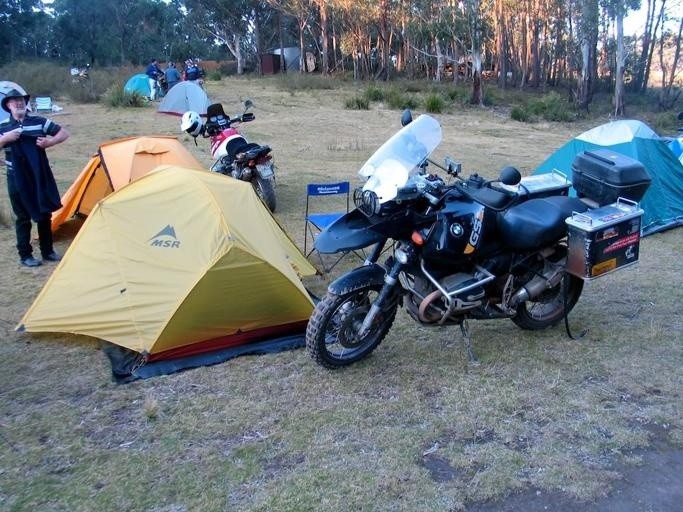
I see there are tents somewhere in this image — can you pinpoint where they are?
[527,119,682,238]
[123,73,158,100]
[50,133,206,237]
[157,80,213,117]
[0,80,31,123]
[10,164,343,384]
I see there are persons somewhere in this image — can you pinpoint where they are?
[144,57,205,101]
[0,89,71,268]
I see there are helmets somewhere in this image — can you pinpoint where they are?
[181,111,203,137]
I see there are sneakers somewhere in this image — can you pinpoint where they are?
[43,254,62,261]
[22,258,42,266]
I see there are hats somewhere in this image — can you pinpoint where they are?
[1,89,30,113]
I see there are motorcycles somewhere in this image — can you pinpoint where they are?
[306,109,650,373]
[71,64,92,86]
[181,99,282,213]
